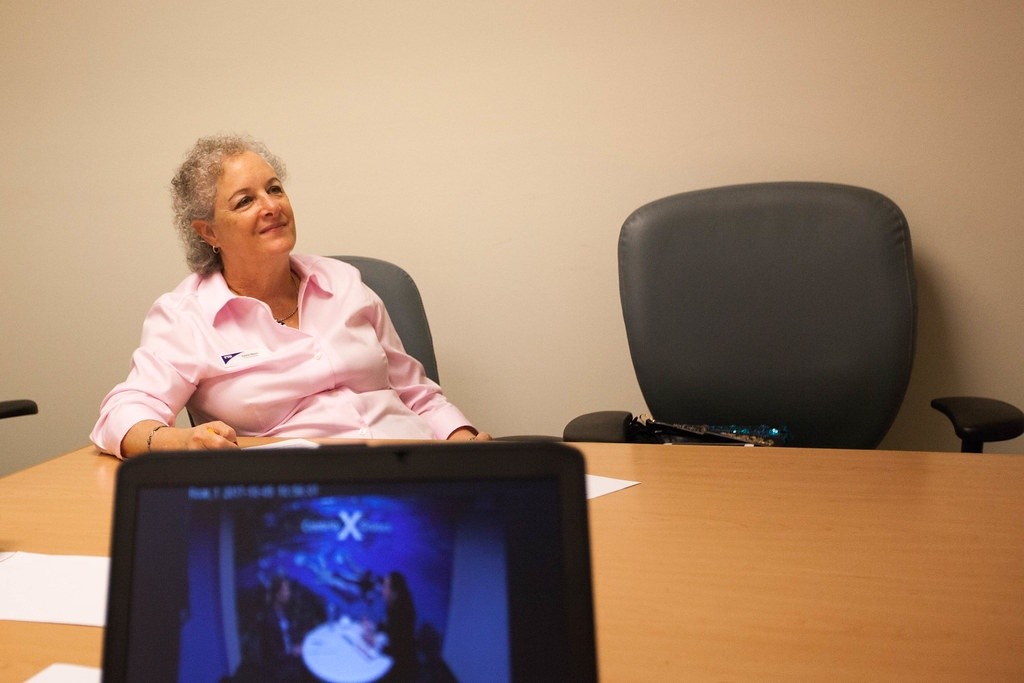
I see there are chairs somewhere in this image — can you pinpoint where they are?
[327,254,441,386]
[563,181,1024,454]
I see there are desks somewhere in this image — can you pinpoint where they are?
[0,443,1024,683]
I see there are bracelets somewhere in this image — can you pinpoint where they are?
[146,425,168,453]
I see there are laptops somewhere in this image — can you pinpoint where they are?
[100,439,600,682]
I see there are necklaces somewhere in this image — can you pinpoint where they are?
[272,306,298,326]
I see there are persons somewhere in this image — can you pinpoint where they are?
[262,576,304,683]
[90,135,493,462]
[366,572,418,682]
